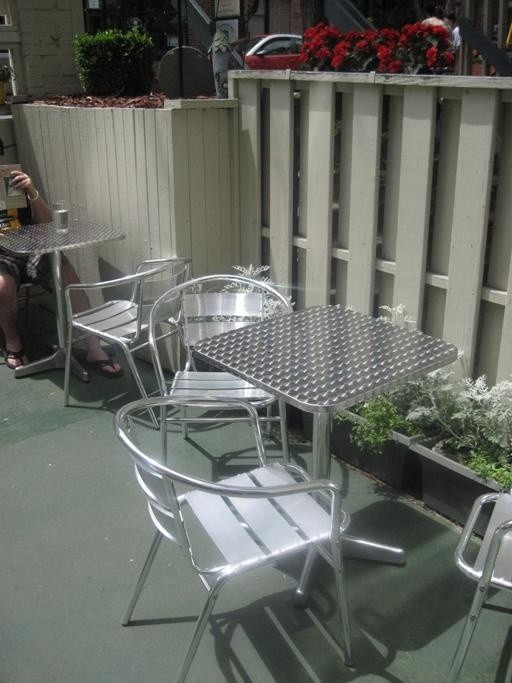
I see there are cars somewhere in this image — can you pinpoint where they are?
[238,33,308,101]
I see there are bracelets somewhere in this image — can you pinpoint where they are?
[27,188,38,201]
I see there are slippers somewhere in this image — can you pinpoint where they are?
[86,354,123,378]
[2,347,28,368]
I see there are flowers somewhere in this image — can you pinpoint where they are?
[299,23,455,73]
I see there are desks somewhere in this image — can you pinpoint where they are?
[191,304,459,612]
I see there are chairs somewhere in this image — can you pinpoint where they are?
[437,491,511,683]
[106,393,356,682]
[61,257,188,426]
[145,272,296,474]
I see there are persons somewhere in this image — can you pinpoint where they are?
[0,137,125,380]
[440,11,462,55]
[420,4,451,48]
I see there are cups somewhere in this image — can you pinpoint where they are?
[52,200,69,235]
